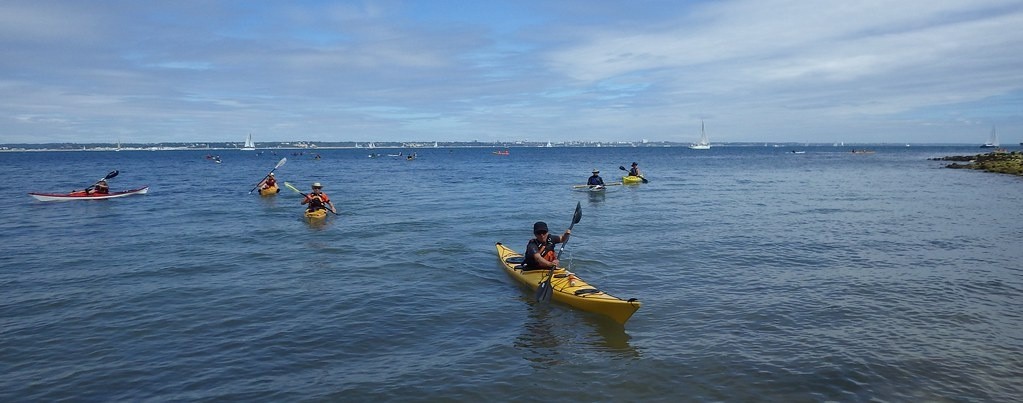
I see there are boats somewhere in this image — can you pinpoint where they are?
[26,184,149,202]
[258,184,280,197]
[216,159,223,165]
[371,155,417,161]
[495,241,644,327]
[303,202,331,223]
[494,152,511,155]
[586,183,609,194]
[623,174,646,185]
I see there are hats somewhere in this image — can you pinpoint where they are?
[312,182,320,186]
[269,173,275,176]
[534,223,548,231]
[631,162,638,165]
[591,168,600,172]
[99,178,106,182]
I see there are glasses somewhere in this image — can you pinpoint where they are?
[536,230,547,234]
[314,187,320,189]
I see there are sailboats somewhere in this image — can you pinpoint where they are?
[239,134,257,152]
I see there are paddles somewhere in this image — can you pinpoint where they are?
[534,200,583,307]
[618,165,649,182]
[573,182,622,189]
[283,182,341,216]
[248,157,287,195]
[72,169,120,193]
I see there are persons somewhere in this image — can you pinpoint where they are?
[588,169,603,185]
[301,183,336,213]
[408,154,412,159]
[399,151,402,156]
[523,222,570,271]
[628,162,639,176]
[95,178,109,194]
[216,156,220,161]
[258,172,276,188]
